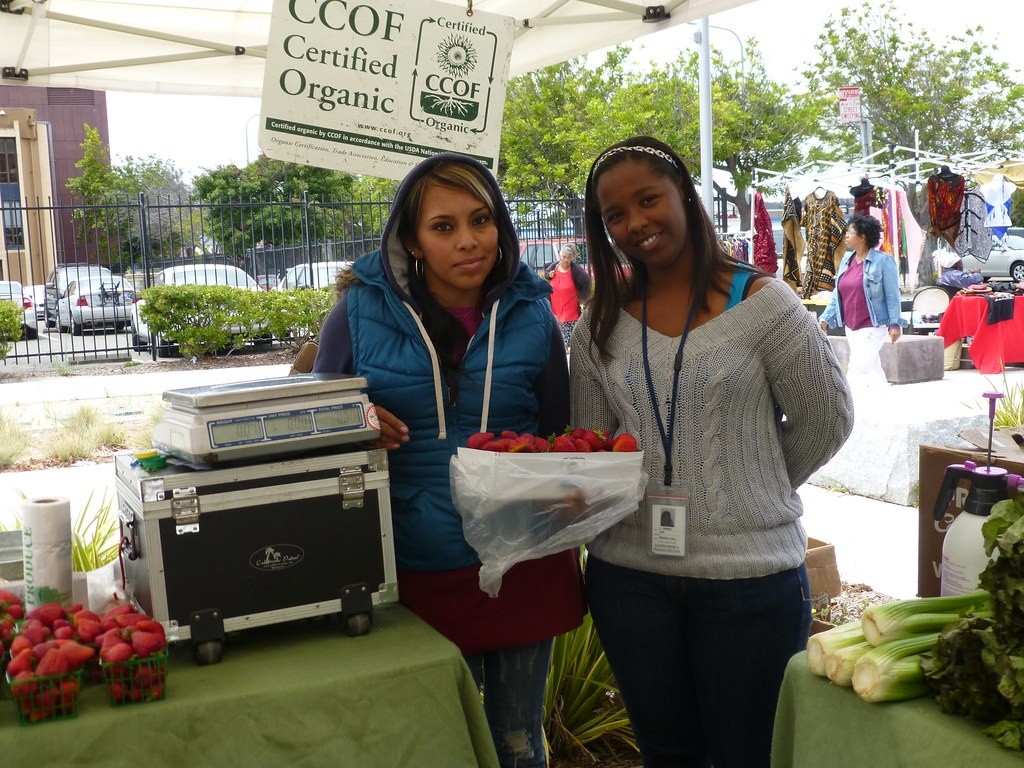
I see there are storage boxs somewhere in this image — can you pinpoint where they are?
[918,425,1024,599]
[113,448,400,667]
[457,446,644,501]
[804,537,841,604]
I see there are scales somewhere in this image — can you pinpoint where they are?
[148,371,382,466]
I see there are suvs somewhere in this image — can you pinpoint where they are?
[43,263,113,329]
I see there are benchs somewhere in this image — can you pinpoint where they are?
[824,334,945,385]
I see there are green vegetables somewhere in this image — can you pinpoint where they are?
[806,499,1024,754]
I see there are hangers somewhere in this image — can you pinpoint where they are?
[815,180,827,192]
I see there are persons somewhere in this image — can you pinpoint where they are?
[819,215,906,393]
[661,511,674,526]
[312,155,590,768]
[937,253,984,290]
[567,138,854,768]
[544,243,591,351]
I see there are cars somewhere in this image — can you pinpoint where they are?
[257,261,356,300]
[519,238,634,280]
[0,281,38,340]
[24,285,45,320]
[54,276,138,336]
[960,227,1024,283]
[130,263,274,358]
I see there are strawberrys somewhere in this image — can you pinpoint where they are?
[465,424,637,452]
[0,590,165,721]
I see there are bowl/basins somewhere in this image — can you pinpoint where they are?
[456,445,644,500]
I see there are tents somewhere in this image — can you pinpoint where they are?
[0,0,757,230]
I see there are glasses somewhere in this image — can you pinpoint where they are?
[846,229,858,236]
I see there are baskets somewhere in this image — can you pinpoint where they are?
[1,604,169,727]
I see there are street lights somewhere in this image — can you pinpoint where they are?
[686,20,744,97]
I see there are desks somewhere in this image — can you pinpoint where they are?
[936,295,1024,374]
[0,600,501,768]
[771,650,1024,768]
